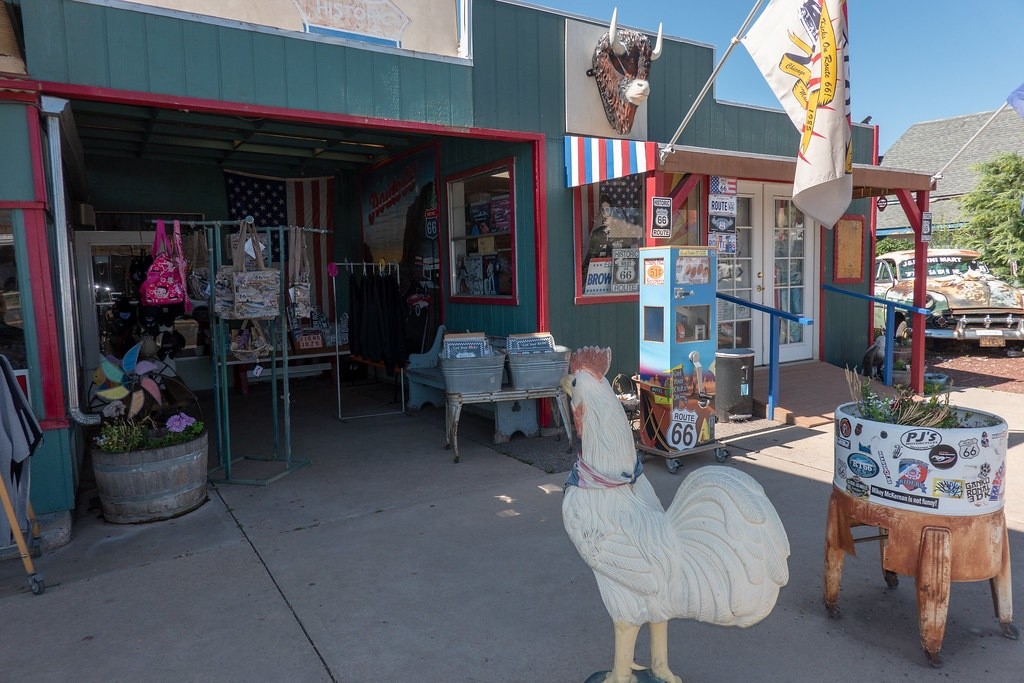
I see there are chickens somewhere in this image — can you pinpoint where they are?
[558,345,790,683]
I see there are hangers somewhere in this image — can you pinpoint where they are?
[363,262,367,276]
[380,263,382,276]
[373,262,375,274]
[393,261,397,271]
[388,262,391,275]
[350,263,354,273]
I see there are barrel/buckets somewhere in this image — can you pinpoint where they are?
[716,349,756,423]
[509,346,571,391]
[438,349,507,393]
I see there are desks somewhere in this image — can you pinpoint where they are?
[215,344,351,398]
[437,385,573,463]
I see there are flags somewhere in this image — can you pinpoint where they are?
[740,0,853,230]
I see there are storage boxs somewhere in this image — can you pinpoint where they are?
[438,350,505,393]
[499,345,571,391]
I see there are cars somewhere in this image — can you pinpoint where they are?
[873,248,1024,348]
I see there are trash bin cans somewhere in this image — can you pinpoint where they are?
[714,349,754,424]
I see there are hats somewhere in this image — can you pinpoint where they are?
[169,330,185,359]
[156,331,178,361]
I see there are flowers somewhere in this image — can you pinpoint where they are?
[166,413,195,433]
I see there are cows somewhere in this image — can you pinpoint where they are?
[592,7,663,136]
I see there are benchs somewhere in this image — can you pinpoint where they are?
[405,325,540,444]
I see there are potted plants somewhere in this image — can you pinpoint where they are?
[833,364,1008,516]
[149,419,150,420]
[892,361,912,387]
[92,421,209,524]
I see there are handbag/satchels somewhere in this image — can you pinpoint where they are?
[229,319,270,360]
[215,220,280,320]
[187,223,211,299]
[140,219,184,306]
[172,220,192,313]
[284,226,312,318]
